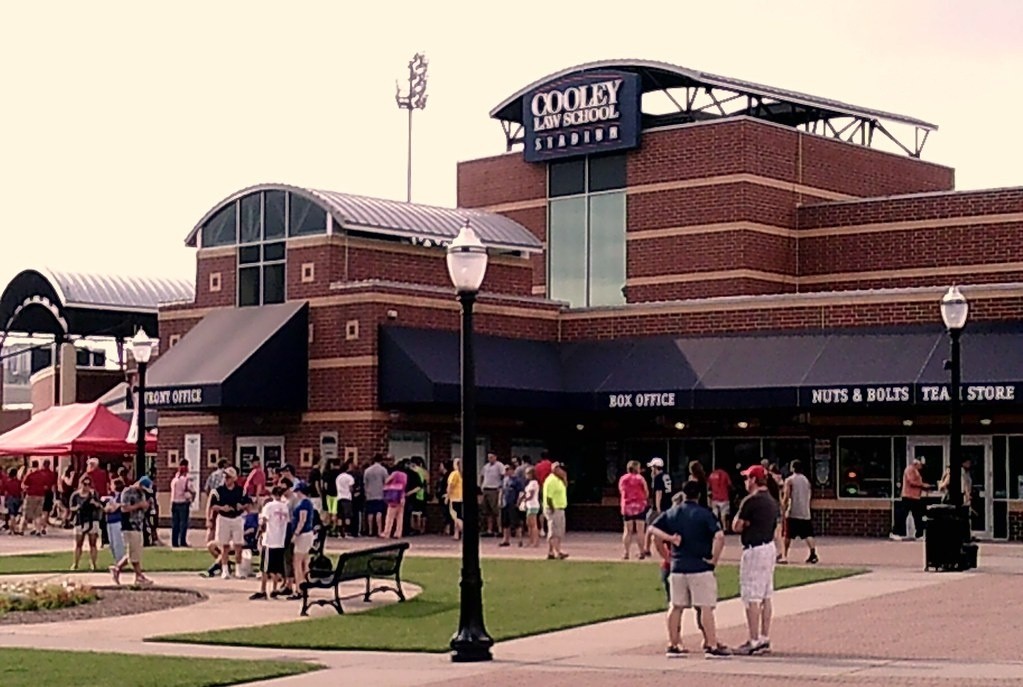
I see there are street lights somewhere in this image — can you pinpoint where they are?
[131,324,154,482]
[444,215,496,663]
[939,279,969,505]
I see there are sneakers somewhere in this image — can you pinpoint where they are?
[732,641,763,655]
[665,645,691,657]
[759,640,771,653]
[134,574,153,587]
[705,647,732,659]
[107,565,120,585]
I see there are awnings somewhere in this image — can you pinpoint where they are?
[378,321,1023,408]
[132,301,310,412]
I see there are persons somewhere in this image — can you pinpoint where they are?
[308,453,463,540]
[68,474,155,585]
[617,457,819,563]
[206,455,326,602]
[478,452,570,558]
[888,458,974,541]
[648,481,734,659]
[731,465,780,656]
[0,456,197,548]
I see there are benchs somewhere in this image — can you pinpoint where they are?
[299,542,410,615]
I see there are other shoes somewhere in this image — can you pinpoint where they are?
[559,553,568,559]
[10,530,96,571]
[172,542,308,601]
[548,554,556,559]
[806,555,818,563]
[499,542,509,546]
[539,529,546,537]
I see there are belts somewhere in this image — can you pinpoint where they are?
[745,541,768,548]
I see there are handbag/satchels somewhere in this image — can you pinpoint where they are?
[183,479,193,501]
[383,471,403,505]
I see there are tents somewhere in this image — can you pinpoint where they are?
[0,401,160,456]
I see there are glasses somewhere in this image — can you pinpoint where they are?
[84,482,91,486]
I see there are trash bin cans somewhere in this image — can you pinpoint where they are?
[925,504,959,567]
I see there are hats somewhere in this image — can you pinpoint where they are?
[740,465,767,479]
[289,481,307,493]
[243,455,259,462]
[646,457,664,467]
[139,476,154,494]
[222,467,237,480]
[682,481,700,496]
